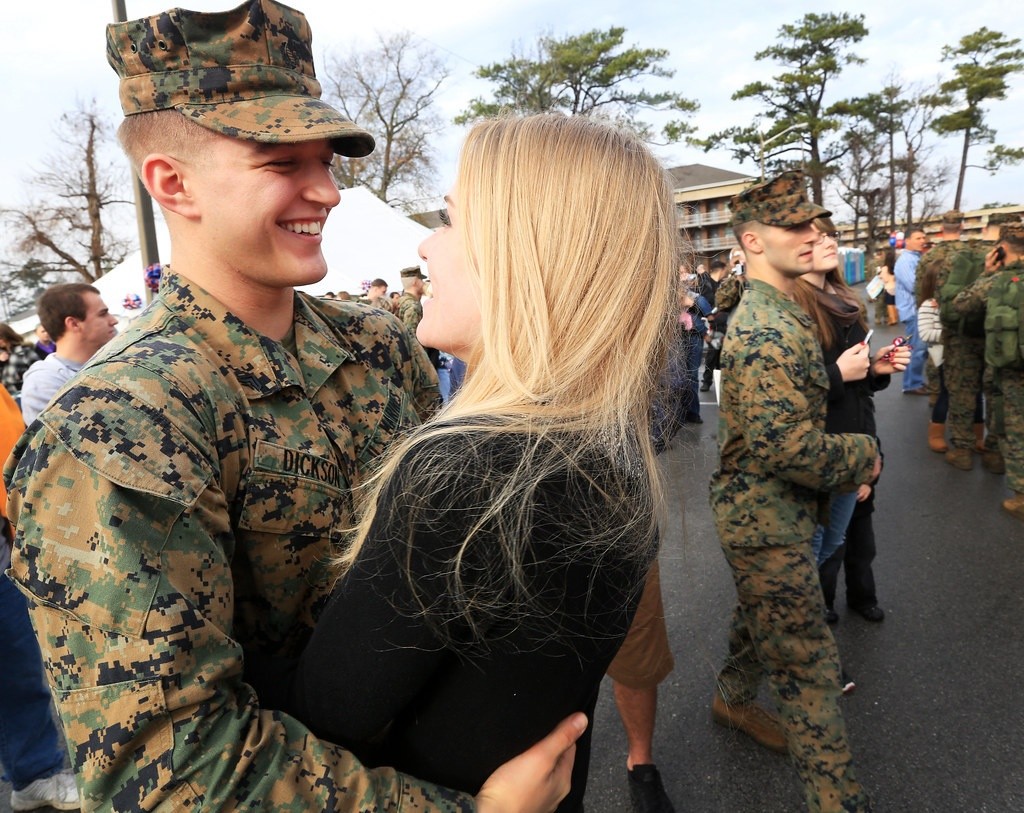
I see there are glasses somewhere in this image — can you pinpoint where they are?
[816,231,841,244]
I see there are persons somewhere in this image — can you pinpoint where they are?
[882,209,1024,520]
[277,114,681,813]
[646,260,727,457]
[0,0,588,813]
[707,170,912,813]
[606,554,674,813]
[324,266,427,335]
[0,283,119,813]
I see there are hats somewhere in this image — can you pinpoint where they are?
[106,0,375,158]
[728,170,832,225]
[943,210,964,223]
[987,214,1022,228]
[992,222,1024,245]
[400,268,427,279]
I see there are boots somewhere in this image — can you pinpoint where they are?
[927,420,948,452]
[974,421,985,454]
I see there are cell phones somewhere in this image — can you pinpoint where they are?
[995,246,1005,262]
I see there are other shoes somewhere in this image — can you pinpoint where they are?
[928,392,938,406]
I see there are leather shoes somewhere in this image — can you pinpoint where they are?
[847,601,884,621]
[922,382,930,389]
[903,384,932,395]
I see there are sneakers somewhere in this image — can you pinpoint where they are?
[945,446,974,472]
[626,764,675,813]
[1003,490,1024,520]
[712,695,788,754]
[982,446,1004,474]
